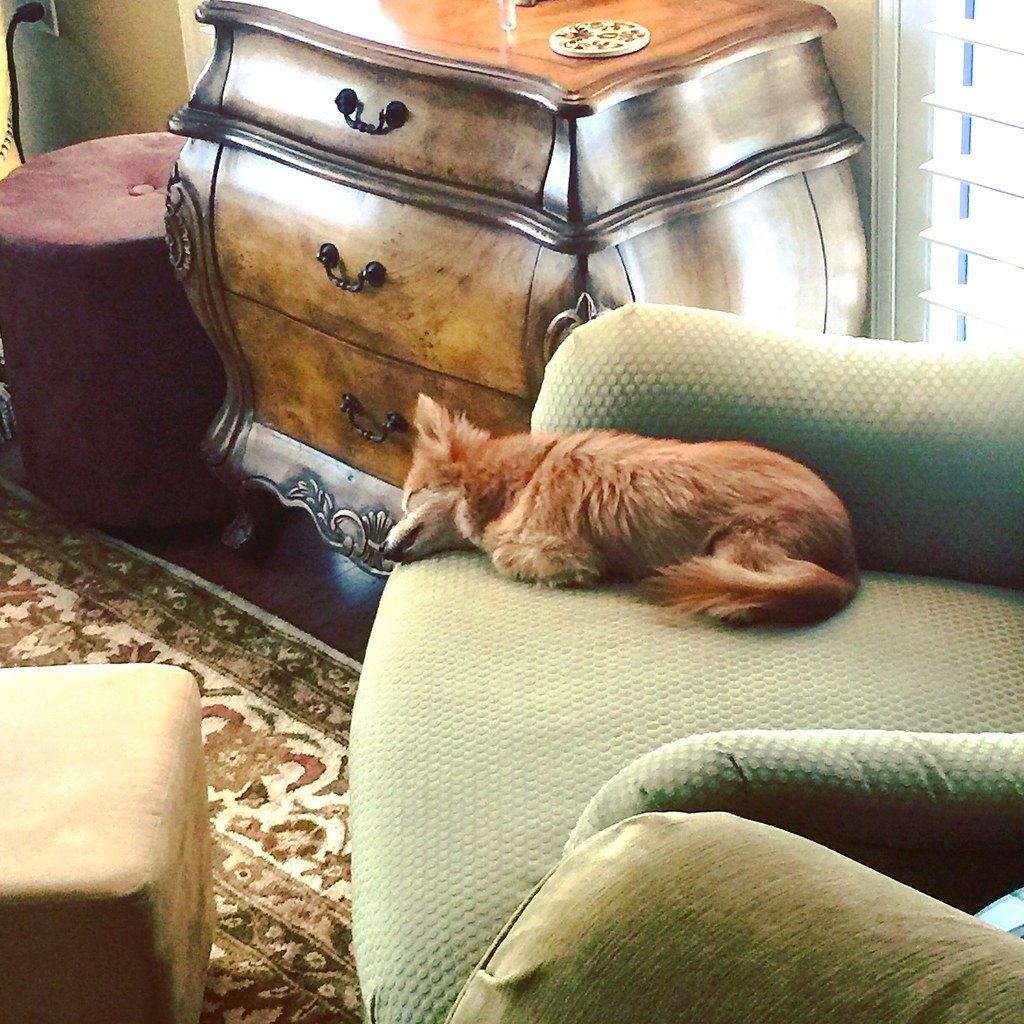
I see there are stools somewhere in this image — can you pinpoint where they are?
[0,664,215,1024]
[0,131,285,527]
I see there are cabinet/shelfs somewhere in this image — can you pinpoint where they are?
[165,0,876,579]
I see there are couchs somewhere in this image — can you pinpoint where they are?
[347,304,1022,1024]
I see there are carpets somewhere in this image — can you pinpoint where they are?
[0,472,360,1024]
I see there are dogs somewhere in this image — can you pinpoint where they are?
[379,390,861,629]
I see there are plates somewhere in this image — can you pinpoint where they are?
[550,20,651,59]
